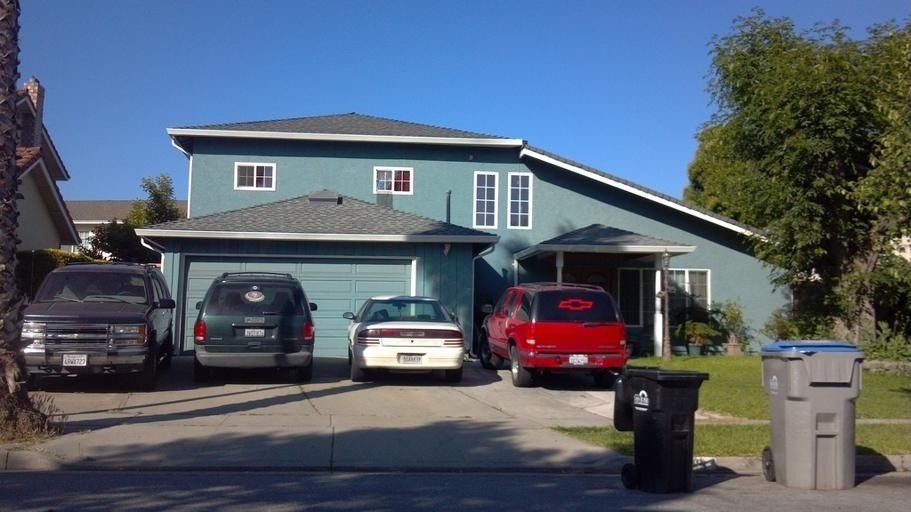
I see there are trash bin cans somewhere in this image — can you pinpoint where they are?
[614,365,709,493]
[760,339,865,490]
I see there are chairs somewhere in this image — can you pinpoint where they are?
[221,292,294,310]
[372,309,389,320]
[416,314,431,321]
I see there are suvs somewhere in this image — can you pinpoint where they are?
[475,280,629,387]
[192,270,318,386]
[16,261,173,386]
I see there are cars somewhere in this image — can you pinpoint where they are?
[342,294,466,382]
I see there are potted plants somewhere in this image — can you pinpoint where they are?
[674,298,745,357]
